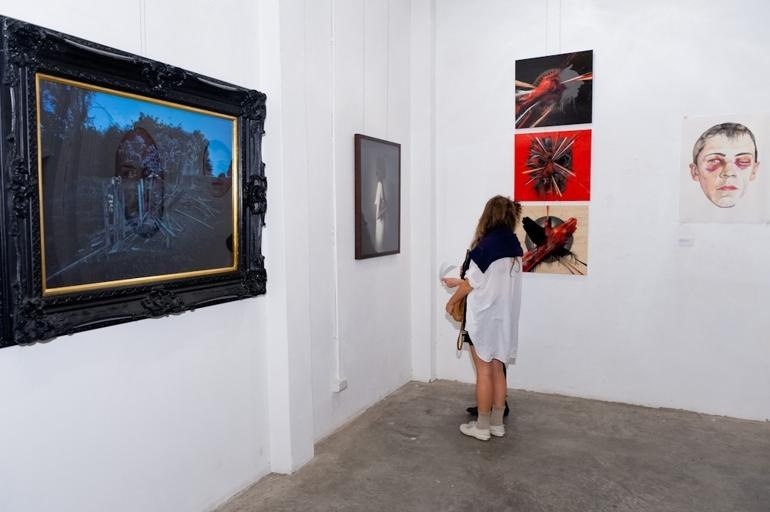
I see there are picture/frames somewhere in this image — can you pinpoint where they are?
[355,134,401,260]
[0,15,267,350]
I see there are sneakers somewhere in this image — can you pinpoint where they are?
[469,420,505,437]
[459,423,491,441]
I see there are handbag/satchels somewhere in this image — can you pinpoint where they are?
[451,296,465,321]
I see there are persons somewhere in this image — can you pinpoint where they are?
[372,154,389,252]
[110,127,168,239]
[444,194,525,442]
[688,122,761,210]
[440,199,521,418]
[201,138,234,199]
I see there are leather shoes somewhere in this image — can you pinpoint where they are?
[466,401,510,416]
